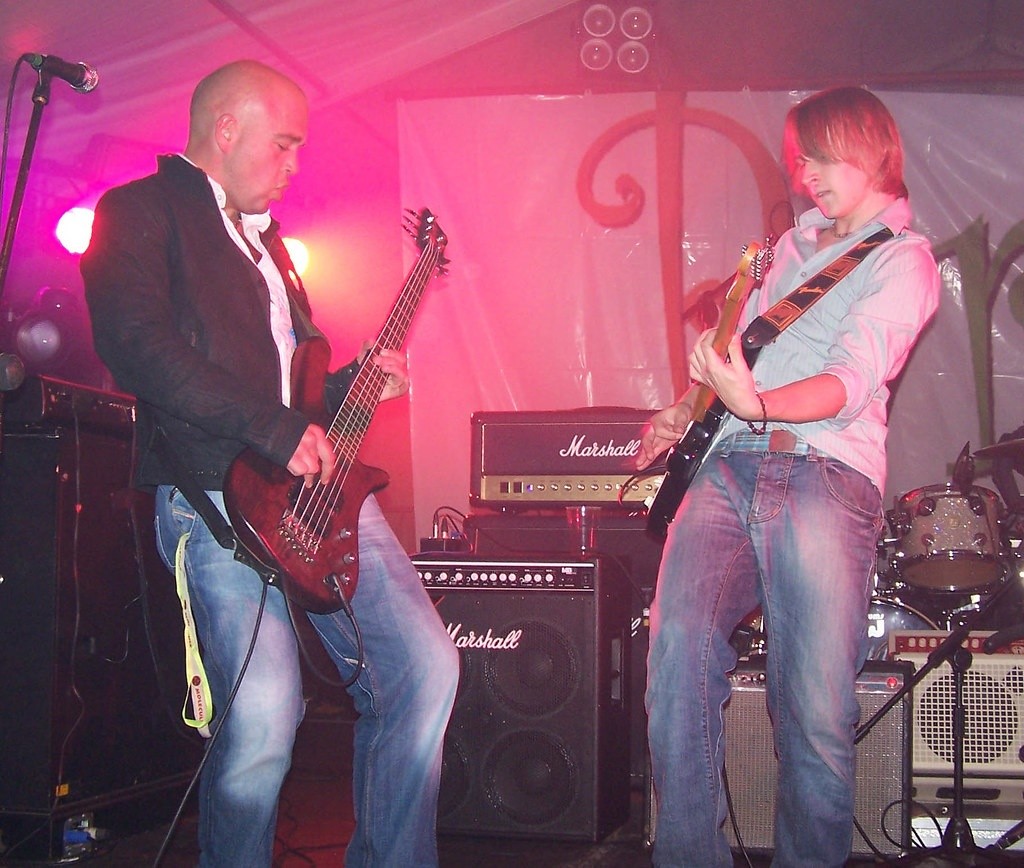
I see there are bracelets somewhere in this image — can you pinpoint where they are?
[747,395,767,435]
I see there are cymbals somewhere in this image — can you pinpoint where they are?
[972,436,1024,458]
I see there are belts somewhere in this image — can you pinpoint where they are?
[712,429,831,459]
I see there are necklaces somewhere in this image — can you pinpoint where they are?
[833,226,851,238]
[235,213,242,227]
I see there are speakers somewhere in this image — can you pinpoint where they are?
[641,658,914,864]
[461,514,667,610]
[410,551,629,842]
[886,628,1024,779]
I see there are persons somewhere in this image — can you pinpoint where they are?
[81,61,459,868]
[636,88,943,868]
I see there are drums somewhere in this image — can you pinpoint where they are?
[889,483,1011,597]
[856,596,942,672]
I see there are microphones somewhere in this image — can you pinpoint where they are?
[22,52,99,92]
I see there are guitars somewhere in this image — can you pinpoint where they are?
[220,204,451,617]
[643,234,774,539]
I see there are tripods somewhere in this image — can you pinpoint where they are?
[854,568,1024,868]
